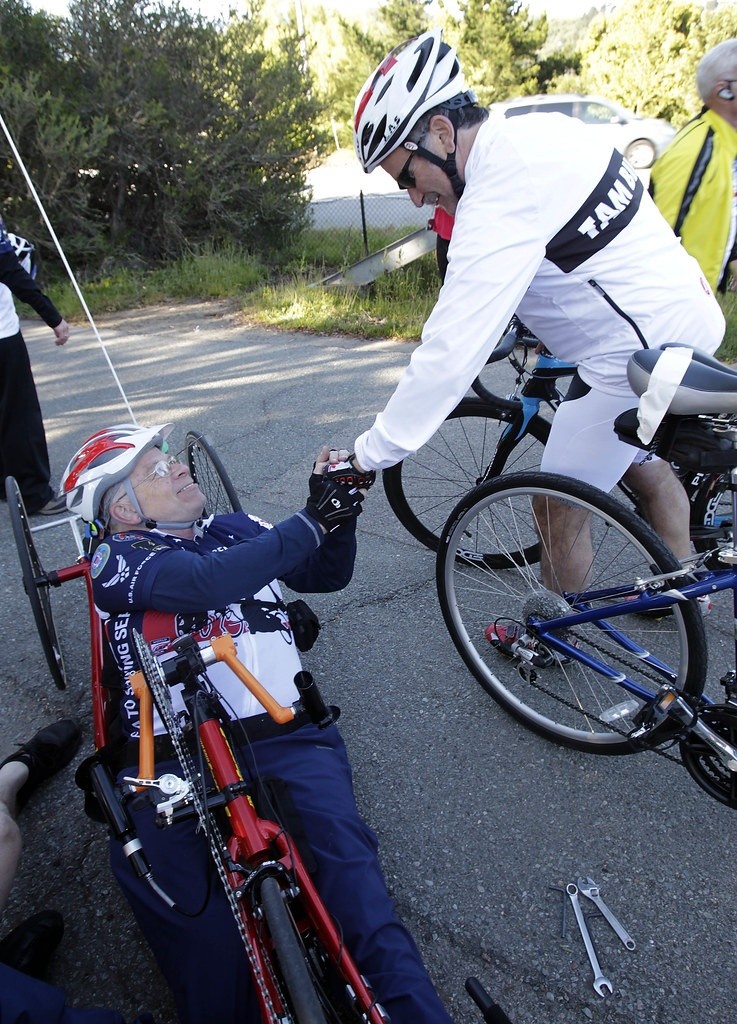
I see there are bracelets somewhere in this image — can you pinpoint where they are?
[344,451,376,490]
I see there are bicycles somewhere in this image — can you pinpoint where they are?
[6,430,510,1023]
[434,342,737,811]
[377,314,737,580]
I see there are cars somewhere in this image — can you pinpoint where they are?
[489,94,679,170]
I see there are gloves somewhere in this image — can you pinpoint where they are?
[322,453,375,492]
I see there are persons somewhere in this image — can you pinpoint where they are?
[1,219,78,522]
[648,37,736,353]
[1,716,132,1024]
[314,29,730,680]
[69,422,457,1024]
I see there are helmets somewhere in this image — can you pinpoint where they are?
[355,26,469,174]
[57,422,179,525]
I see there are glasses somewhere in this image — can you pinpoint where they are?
[115,454,185,503]
[397,123,431,190]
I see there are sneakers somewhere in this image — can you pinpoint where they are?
[38,490,68,515]
[484,621,575,667]
[0,909,64,980]
[0,719,80,819]
[623,588,713,620]
